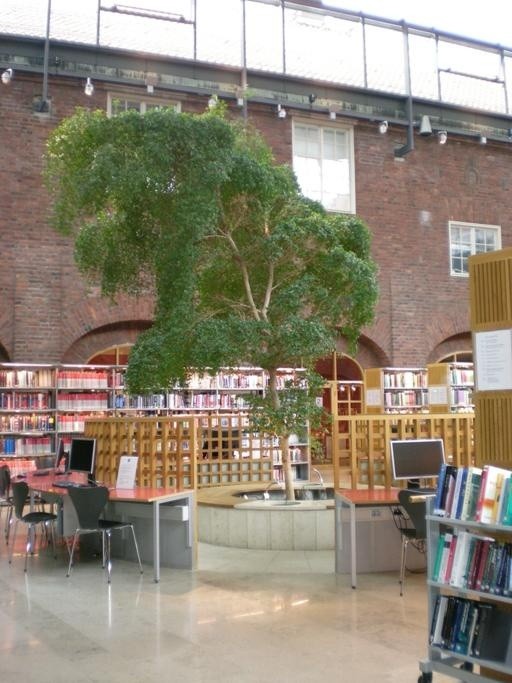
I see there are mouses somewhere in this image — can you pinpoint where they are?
[17,474,26,478]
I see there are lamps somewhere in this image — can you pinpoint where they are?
[0,66,489,147]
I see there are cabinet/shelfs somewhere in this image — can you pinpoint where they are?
[467,248,512,543]
[428,361,475,413]
[0,361,311,503]
[417,496,512,683]
[363,365,427,426]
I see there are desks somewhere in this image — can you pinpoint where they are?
[10,469,195,583]
[333,488,436,590]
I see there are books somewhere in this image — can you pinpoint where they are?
[57,368,113,468]
[1,368,57,489]
[384,368,475,426]
[211,418,305,480]
[114,368,305,417]
[426,462,512,660]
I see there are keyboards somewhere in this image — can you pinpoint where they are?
[33,469,49,476]
[52,480,92,488]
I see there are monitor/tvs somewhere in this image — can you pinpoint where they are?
[55,437,72,475]
[67,438,97,484]
[202,430,239,459]
[390,438,445,490]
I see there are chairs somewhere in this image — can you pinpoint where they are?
[0,465,145,584]
[399,490,437,596]
